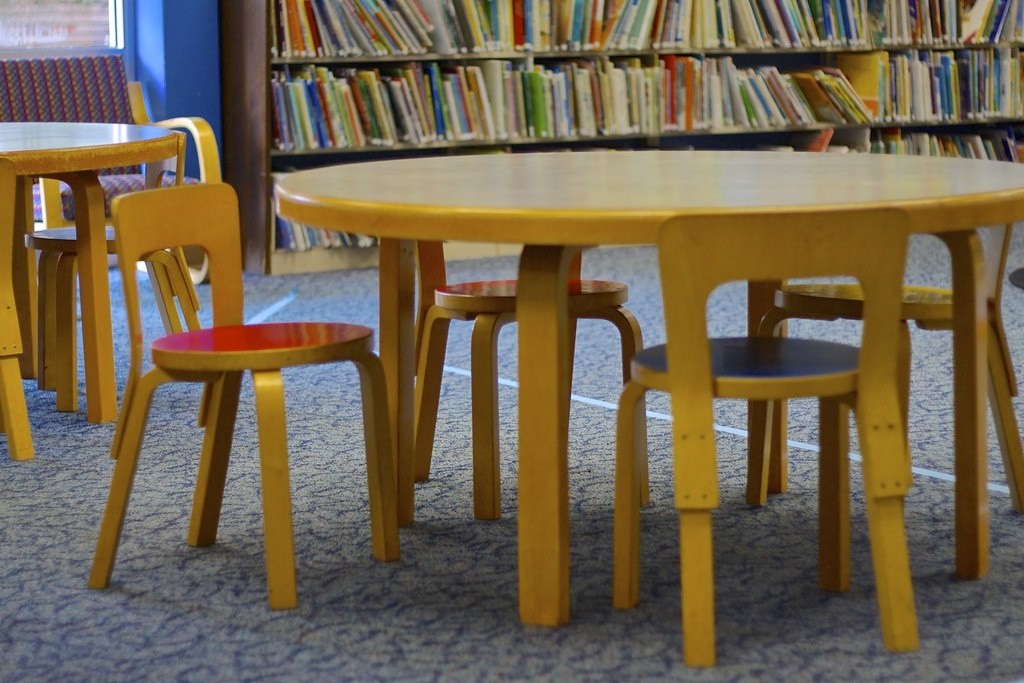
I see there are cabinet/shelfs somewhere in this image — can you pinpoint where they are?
[220,0,1024,275]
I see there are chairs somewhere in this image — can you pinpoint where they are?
[88,181,402,611]
[746,225,1024,513]
[613,206,921,653]
[25,131,202,425]
[415,238,649,522]
[0,159,34,462]
[0,54,222,284]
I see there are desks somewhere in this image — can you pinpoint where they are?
[0,122,179,426]
[274,150,1024,628]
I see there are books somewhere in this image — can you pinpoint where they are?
[268,0,1024,251]
